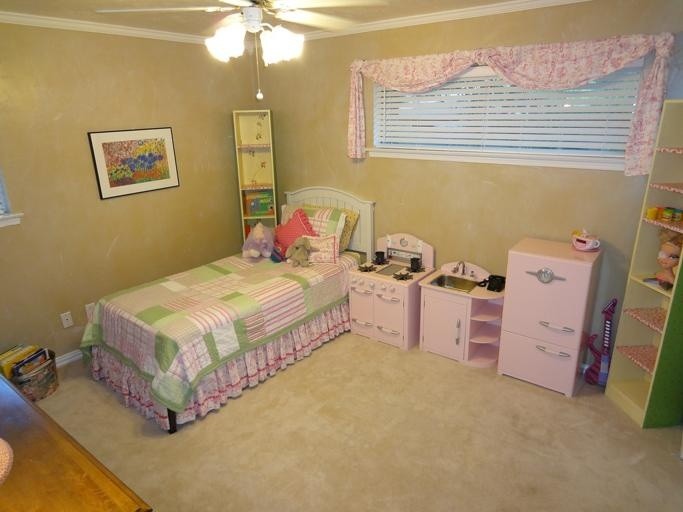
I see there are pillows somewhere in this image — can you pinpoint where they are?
[270,200,362,266]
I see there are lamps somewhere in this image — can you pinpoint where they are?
[199,5,310,67]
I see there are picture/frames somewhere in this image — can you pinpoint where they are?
[86,124,182,199]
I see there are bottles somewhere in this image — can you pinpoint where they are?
[645,206,681,224]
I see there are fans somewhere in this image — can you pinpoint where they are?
[95,0,390,36]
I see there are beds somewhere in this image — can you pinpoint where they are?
[81,185,376,435]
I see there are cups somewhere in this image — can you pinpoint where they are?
[410,257,420,271]
[488,274,504,292]
[375,252,385,264]
[572,235,601,250]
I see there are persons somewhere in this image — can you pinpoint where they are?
[657,227,683,292]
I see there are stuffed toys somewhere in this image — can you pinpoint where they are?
[241,221,276,258]
[285,236,311,267]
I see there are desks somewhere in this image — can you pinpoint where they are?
[0,373,155,512]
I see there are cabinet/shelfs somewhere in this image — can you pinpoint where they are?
[496,234,607,400]
[605,97,682,431]
[345,232,435,351]
[415,268,504,368]
[230,108,279,250]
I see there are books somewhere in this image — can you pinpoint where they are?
[0,344,50,379]
